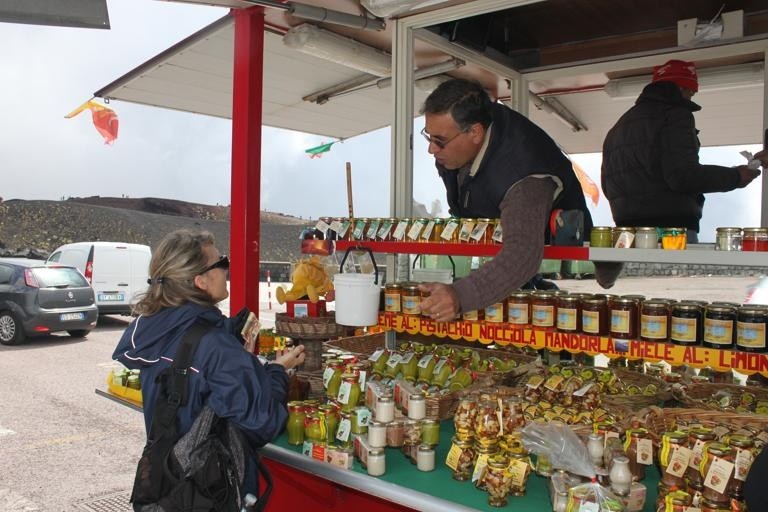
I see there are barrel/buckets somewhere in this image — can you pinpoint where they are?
[410,254,456,284]
[333,246,382,327]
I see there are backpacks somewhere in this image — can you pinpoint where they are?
[127,319,272,511]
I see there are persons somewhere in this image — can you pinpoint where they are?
[113,229,306,512]
[415,77,595,324]
[600,60,761,244]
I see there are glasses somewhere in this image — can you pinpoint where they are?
[199,254,230,275]
[421,123,469,149]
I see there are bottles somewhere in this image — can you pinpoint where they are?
[551,289,768,308]
[524,369,550,402]
[448,366,474,393]
[484,300,506,323]
[371,348,388,376]
[461,308,484,322]
[492,219,505,245]
[475,219,496,245]
[611,456,630,497]
[628,431,646,483]
[454,396,479,444]
[127,375,140,390]
[321,348,372,364]
[716,227,742,251]
[669,306,704,346]
[451,437,474,482]
[424,422,440,447]
[532,405,627,442]
[390,423,406,448]
[394,218,415,242]
[540,372,568,404]
[344,366,368,404]
[339,373,360,415]
[635,226,659,249]
[506,291,531,325]
[605,437,623,486]
[699,499,745,512]
[607,299,638,340]
[508,455,527,500]
[661,227,687,250]
[416,353,438,387]
[535,451,552,479]
[324,362,343,402]
[485,454,513,507]
[701,443,735,503]
[337,218,357,241]
[370,424,386,449]
[308,411,326,444]
[376,219,398,242]
[410,218,430,242]
[368,381,394,423]
[655,485,692,511]
[503,400,527,455]
[736,309,768,354]
[401,280,422,315]
[417,447,436,472]
[352,436,368,465]
[475,396,501,454]
[443,218,463,244]
[580,297,607,337]
[658,431,688,489]
[130,369,140,375]
[742,228,768,251]
[571,381,604,405]
[612,225,633,247]
[406,445,420,460]
[729,435,759,496]
[401,351,418,383]
[318,404,338,445]
[704,304,737,350]
[407,422,422,447]
[259,327,275,355]
[459,217,479,244]
[395,386,428,420]
[471,449,494,491]
[590,227,613,247]
[556,375,585,403]
[586,434,604,465]
[531,292,556,328]
[303,399,319,410]
[316,218,336,241]
[431,356,454,389]
[353,217,372,240]
[556,296,581,332]
[367,451,387,478]
[688,428,716,489]
[348,408,370,435]
[288,401,307,445]
[640,301,670,343]
[383,353,401,379]
[419,282,445,321]
[707,389,768,409]
[429,218,447,242]
[385,282,402,313]
[328,217,344,241]
[366,218,387,242]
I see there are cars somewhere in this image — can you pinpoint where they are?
[1,258,99,345]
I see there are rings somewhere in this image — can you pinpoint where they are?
[437,313,439,318]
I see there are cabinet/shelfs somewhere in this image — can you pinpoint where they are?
[94,242,768,512]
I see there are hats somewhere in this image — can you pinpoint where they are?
[652,61,698,93]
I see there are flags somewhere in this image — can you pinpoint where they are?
[64,100,118,145]
[304,143,333,159]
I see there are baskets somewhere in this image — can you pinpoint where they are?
[321,333,540,387]
[646,408,768,451]
[571,366,671,410]
[674,383,768,413]
[426,371,502,420]
[296,370,324,395]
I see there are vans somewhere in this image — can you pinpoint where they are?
[47,241,152,319]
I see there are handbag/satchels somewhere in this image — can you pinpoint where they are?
[131,323,241,511]
[275,313,347,337]
